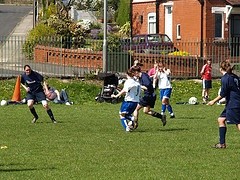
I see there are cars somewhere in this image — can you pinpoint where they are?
[123,33,175,54]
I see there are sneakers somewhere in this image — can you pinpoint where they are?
[202,97,207,104]
[161,115,167,125]
[52,119,56,123]
[126,122,137,131]
[170,113,175,118]
[212,143,226,148]
[31,117,37,123]
[161,112,165,115]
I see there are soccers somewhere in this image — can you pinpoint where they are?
[128,121,138,130]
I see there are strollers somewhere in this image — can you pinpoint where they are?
[96,71,121,103]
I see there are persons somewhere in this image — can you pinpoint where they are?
[156,62,175,118]
[20,64,74,123]
[111,60,167,132]
[201,57,213,104]
[206,60,240,148]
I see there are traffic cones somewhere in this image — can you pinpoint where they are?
[9,74,22,103]
